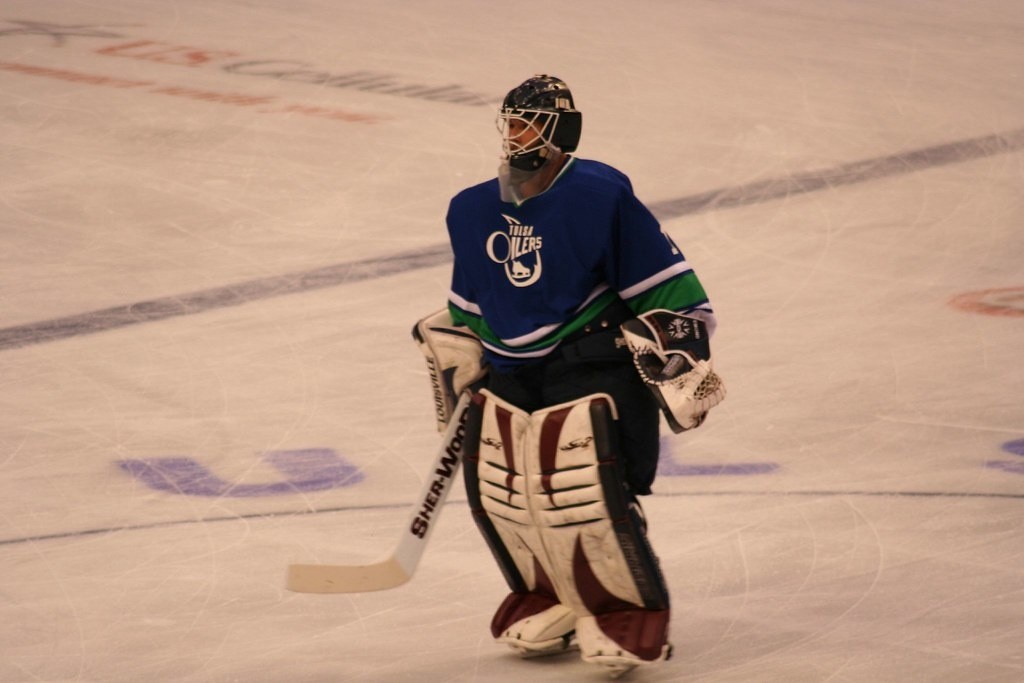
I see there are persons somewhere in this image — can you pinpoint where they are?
[412,74,723,678]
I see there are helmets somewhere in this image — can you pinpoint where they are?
[494,75,583,172]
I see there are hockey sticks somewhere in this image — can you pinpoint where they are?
[280,385,481,598]
